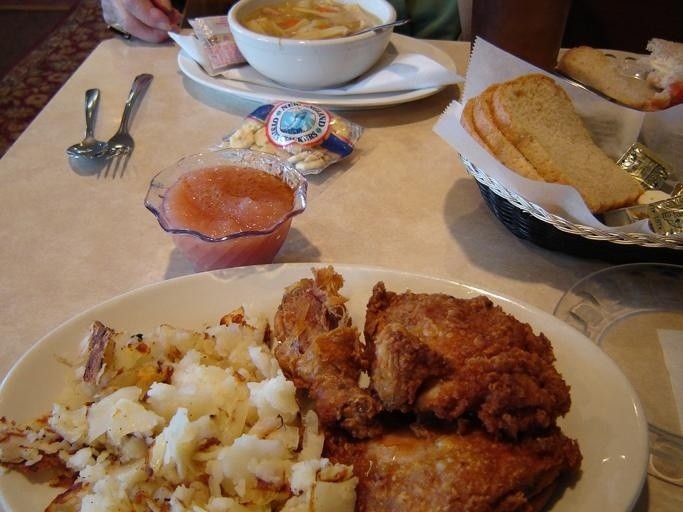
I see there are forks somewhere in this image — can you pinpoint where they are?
[101,74,151,159]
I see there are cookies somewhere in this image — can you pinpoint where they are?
[219,115,350,170]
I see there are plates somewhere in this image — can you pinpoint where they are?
[553,262,683,462]
[175,31,457,114]
[0,258,649,511]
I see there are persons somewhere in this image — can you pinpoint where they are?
[101,0,475,42]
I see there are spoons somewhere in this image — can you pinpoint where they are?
[68,88,107,157]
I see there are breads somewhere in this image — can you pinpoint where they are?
[461,37,682,218]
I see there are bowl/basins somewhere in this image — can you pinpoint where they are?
[143,151,313,269]
[226,2,400,95]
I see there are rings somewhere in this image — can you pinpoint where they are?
[112,24,127,33]
